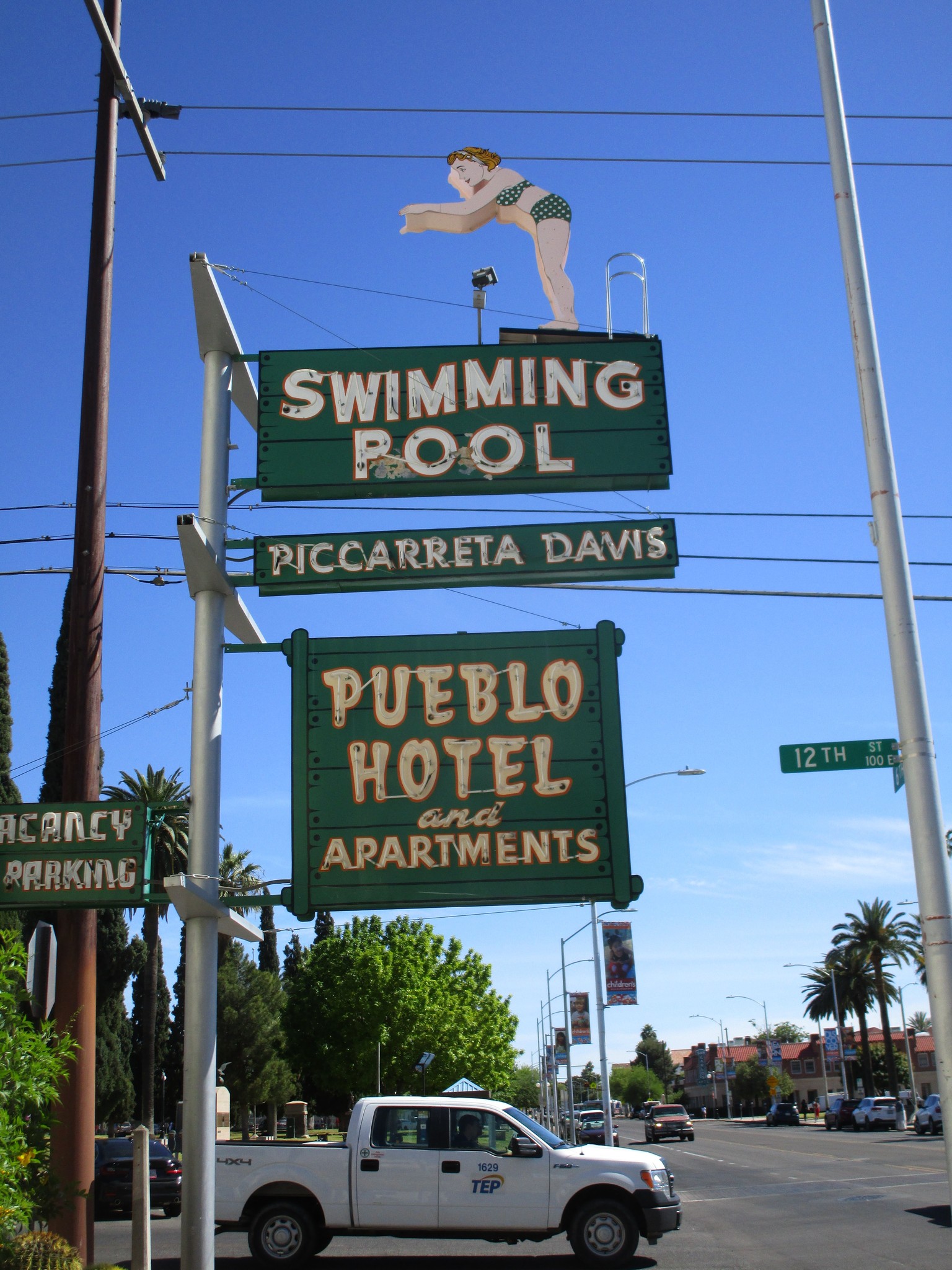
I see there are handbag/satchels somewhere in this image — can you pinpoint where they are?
[702,1111,703,1114]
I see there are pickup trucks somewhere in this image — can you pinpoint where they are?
[215,1096,684,1270]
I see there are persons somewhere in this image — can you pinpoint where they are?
[336,1117,339,1128]
[700,1105,707,1118]
[801,1099,810,1121]
[895,1097,906,1131]
[452,1114,483,1148]
[813,1097,820,1123]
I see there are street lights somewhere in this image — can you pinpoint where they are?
[560,908,640,1145]
[536,1010,571,1128]
[782,962,849,1101]
[159,1070,167,1139]
[572,1081,583,1104]
[589,766,705,1147]
[897,982,919,1106]
[689,1014,731,1119]
[546,957,602,1139]
[726,995,776,1104]
[657,1040,668,1105]
[584,1074,599,1100]
[625,1050,651,1102]
[706,1071,717,1119]
[577,1078,589,1101]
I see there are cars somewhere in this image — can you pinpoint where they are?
[17,1136,183,1218]
[532,1099,660,1147]
[106,1122,133,1138]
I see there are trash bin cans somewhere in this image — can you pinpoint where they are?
[714,1113,720,1119]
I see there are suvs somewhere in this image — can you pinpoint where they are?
[824,1099,863,1131]
[913,1094,943,1136]
[766,1102,800,1127]
[644,1104,695,1144]
[851,1096,898,1133]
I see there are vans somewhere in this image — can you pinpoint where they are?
[808,1092,848,1113]
